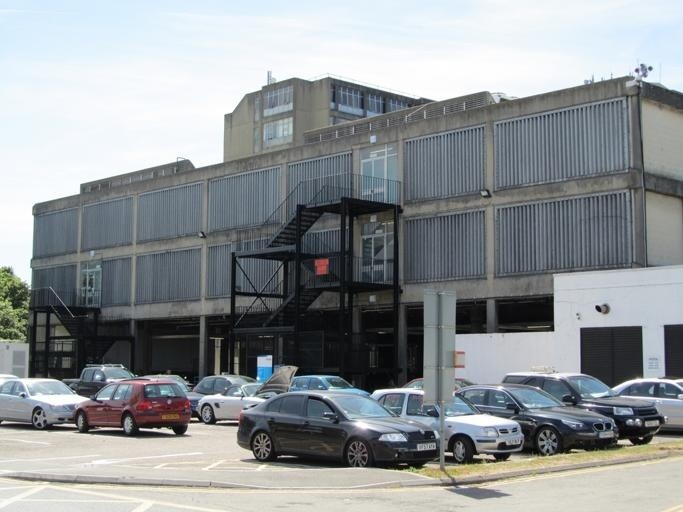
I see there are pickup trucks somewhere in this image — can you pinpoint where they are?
[60,362,136,396]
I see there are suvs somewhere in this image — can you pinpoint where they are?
[502,370,668,448]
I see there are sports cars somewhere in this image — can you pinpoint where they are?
[194,364,299,425]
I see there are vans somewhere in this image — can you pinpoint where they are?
[366,387,528,466]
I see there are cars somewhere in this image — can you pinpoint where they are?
[140,373,205,421]
[70,377,193,437]
[236,387,440,469]
[0,376,91,431]
[453,381,617,457]
[386,375,477,401]
[582,377,683,434]
[0,373,19,385]
[289,374,371,397]
[191,372,262,396]
